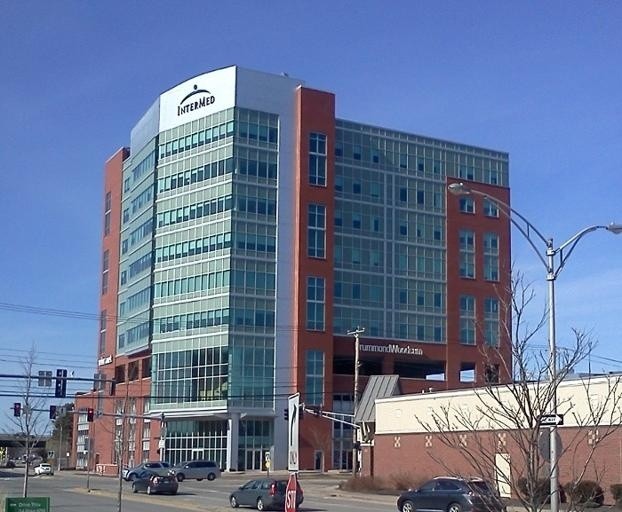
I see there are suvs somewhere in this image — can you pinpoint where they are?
[230,479,304,511]
[123,461,172,482]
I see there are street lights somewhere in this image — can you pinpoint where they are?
[445,182,622,512]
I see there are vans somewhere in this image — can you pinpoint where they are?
[170,461,220,481]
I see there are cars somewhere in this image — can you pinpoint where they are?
[131,471,178,494]
[396,474,506,511]
[34,463,53,475]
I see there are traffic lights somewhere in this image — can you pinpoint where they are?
[86,407,94,422]
[283,408,288,420]
[14,403,20,417]
[48,405,56,420]
